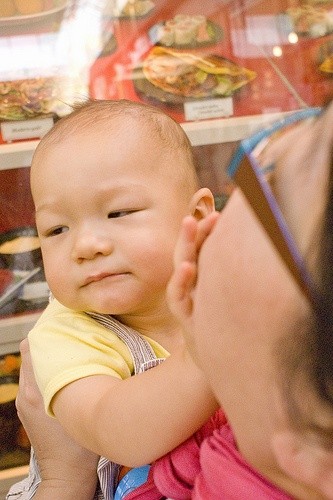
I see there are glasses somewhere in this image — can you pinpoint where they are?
[225,108,315,310]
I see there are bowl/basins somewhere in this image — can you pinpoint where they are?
[0,226,44,272]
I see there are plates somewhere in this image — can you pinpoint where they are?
[0,267,40,310]
[146,19,223,49]
[134,44,242,106]
[17,280,53,305]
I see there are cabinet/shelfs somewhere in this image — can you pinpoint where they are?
[0,2,333,500]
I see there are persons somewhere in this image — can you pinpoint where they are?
[13,93,333,500]
[0,99,223,500]
